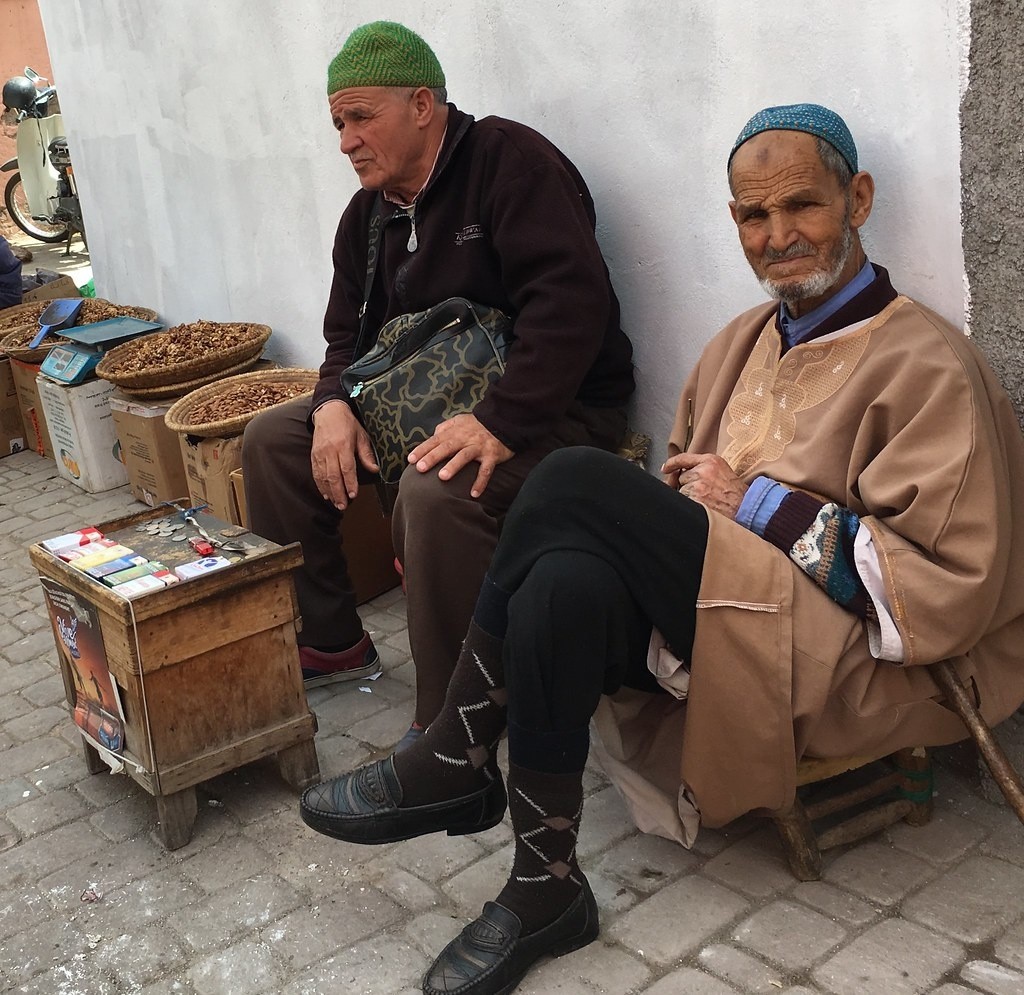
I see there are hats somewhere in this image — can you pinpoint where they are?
[728,104,859,175]
[327,21,445,95]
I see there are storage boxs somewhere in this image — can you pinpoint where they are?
[178,431,247,528]
[22,268,82,305]
[35,373,129,493]
[109,393,190,507]
[0,353,28,458]
[10,356,56,461]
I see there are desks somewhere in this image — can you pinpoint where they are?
[30,498,322,852]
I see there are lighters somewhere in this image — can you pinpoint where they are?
[187,533,216,556]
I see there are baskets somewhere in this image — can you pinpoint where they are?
[0,298,157,363]
[164,367,319,437]
[96,322,271,401]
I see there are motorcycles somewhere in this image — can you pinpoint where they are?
[0,66,89,258]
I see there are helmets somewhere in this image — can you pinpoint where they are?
[3,76,36,109]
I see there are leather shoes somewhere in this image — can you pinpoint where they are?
[422,873,600,995]
[300,751,508,845]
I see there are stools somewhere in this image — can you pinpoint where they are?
[772,741,934,880]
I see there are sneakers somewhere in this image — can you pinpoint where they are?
[299,630,381,690]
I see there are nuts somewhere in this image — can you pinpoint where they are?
[0,297,150,349]
[111,318,260,374]
[187,381,308,425]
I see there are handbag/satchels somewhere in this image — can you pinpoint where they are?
[340,297,517,487]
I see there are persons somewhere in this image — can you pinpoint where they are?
[294,97,1023,994]
[238,16,639,762]
